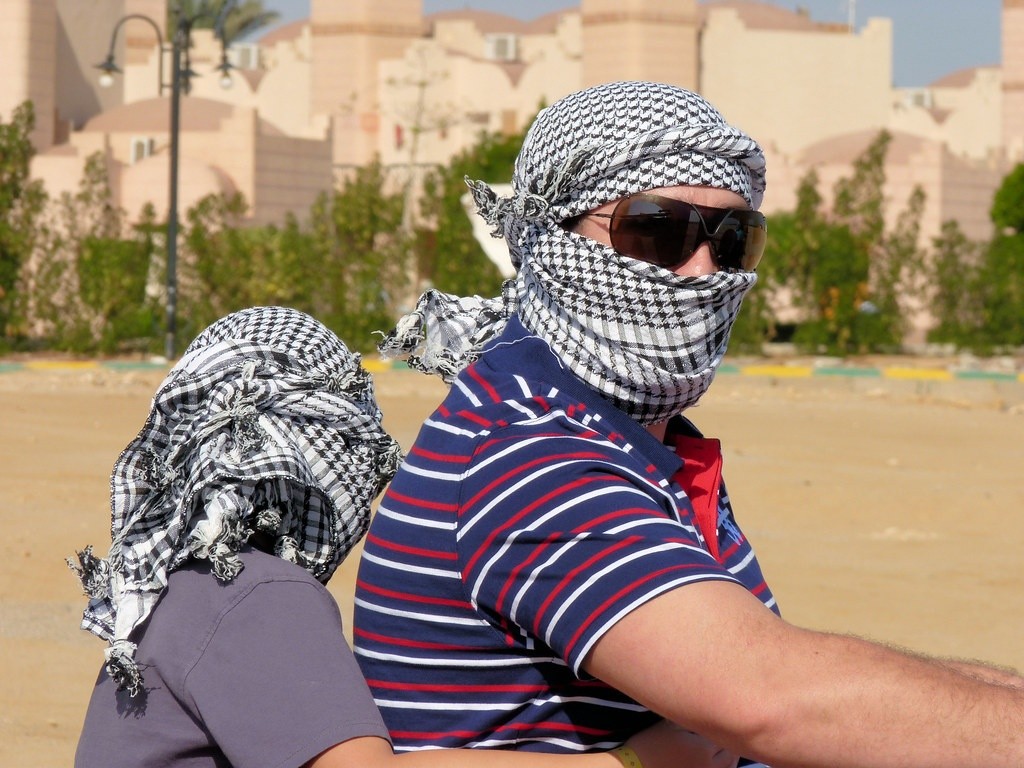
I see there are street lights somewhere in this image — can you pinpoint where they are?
[94,11,236,368]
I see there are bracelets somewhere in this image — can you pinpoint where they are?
[610,745,642,768]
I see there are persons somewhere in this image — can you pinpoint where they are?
[63,305,741,768]
[352,80,1024,768]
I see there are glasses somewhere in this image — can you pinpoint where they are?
[577,193,770,276]
